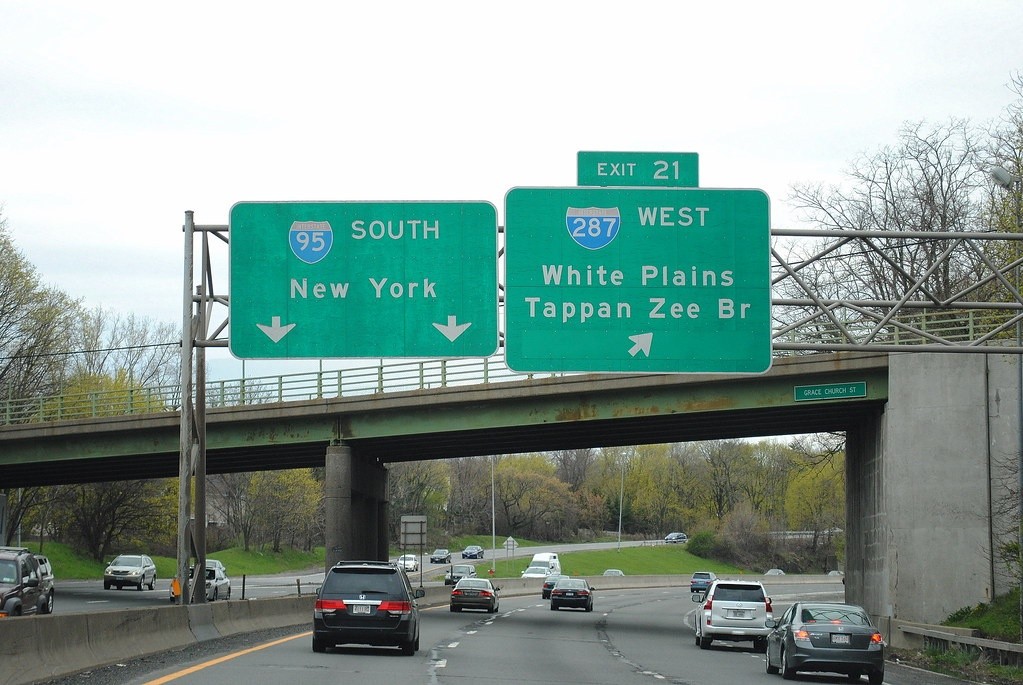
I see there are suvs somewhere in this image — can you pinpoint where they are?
[693,580,774,652]
[311,560,425,656]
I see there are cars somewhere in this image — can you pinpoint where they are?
[430,549,452,564]
[764,600,885,685]
[398,554,419,572]
[104,553,157,592]
[444,564,478,585]
[765,568,785,579]
[542,575,569,598]
[690,572,717,593]
[449,577,500,614]
[665,532,687,543]
[550,579,595,612]
[169,560,231,601]
[462,545,484,560]
[828,570,844,576]
[603,569,624,577]
[520,552,561,578]
[0,546,56,616]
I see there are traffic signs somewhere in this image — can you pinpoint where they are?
[227,201,497,360]
[504,188,772,376]
